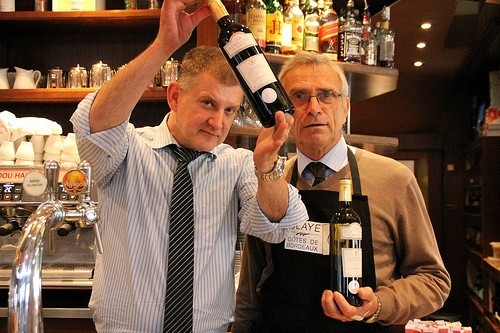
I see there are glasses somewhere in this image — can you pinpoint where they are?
[289,91,343,106]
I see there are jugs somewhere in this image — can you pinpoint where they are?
[0,67,42,89]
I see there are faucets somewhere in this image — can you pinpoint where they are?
[8,198,67,333]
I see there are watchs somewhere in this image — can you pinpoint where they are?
[362,297,381,324]
[254,157,285,182]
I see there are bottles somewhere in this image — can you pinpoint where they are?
[330,177,364,309]
[207,0,296,129]
[228,0,396,71]
[45,56,179,88]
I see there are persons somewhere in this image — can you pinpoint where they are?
[71,1,309,333]
[235,52,451,333]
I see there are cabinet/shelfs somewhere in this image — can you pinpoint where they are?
[0,0,212,189]
[224,52,397,160]
[461,15,500,333]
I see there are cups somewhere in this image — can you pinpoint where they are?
[490,242,500,258]
[0,132,82,168]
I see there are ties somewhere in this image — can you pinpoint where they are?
[306,162,326,187]
[163,145,204,333]
